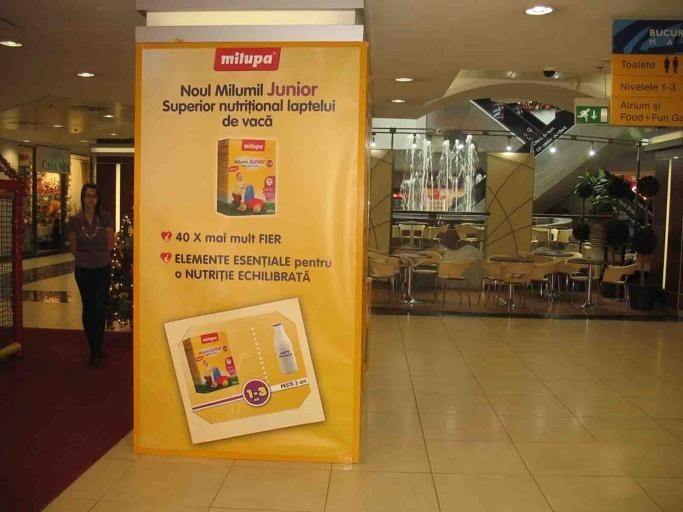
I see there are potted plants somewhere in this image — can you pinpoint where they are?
[572,169,660,311]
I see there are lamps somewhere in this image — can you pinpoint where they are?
[371,126,643,157]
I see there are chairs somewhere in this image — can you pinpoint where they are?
[366,223,642,315]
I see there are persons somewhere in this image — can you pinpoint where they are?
[65,182,115,367]
[230,172,246,205]
[201,360,214,385]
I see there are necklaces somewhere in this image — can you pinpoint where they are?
[80,211,99,239]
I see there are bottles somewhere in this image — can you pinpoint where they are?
[272,321,300,377]
